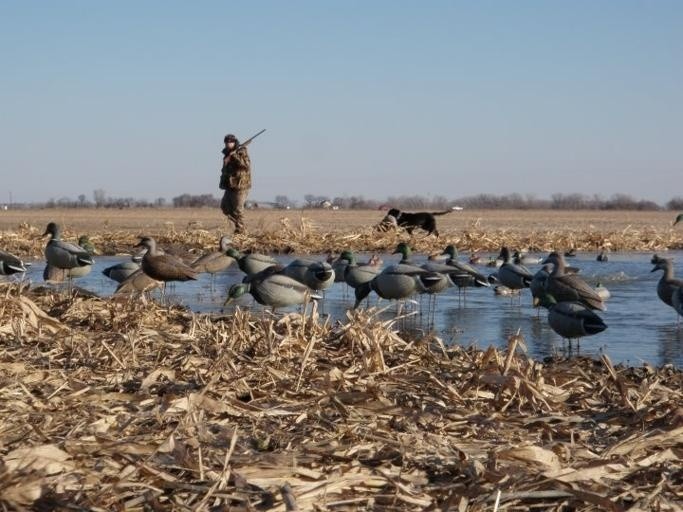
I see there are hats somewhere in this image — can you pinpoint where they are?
[223,134,237,143]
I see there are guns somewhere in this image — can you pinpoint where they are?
[224,129,267,167]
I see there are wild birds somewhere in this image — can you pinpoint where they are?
[650,258,683,322]
[101,234,612,321]
[673,214,683,226]
[43,223,95,285]
[0,249,28,275]
[534,291,608,347]
[651,253,675,264]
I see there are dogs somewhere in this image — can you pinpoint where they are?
[387,208,453,239]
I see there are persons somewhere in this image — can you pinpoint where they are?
[218,134,253,236]
